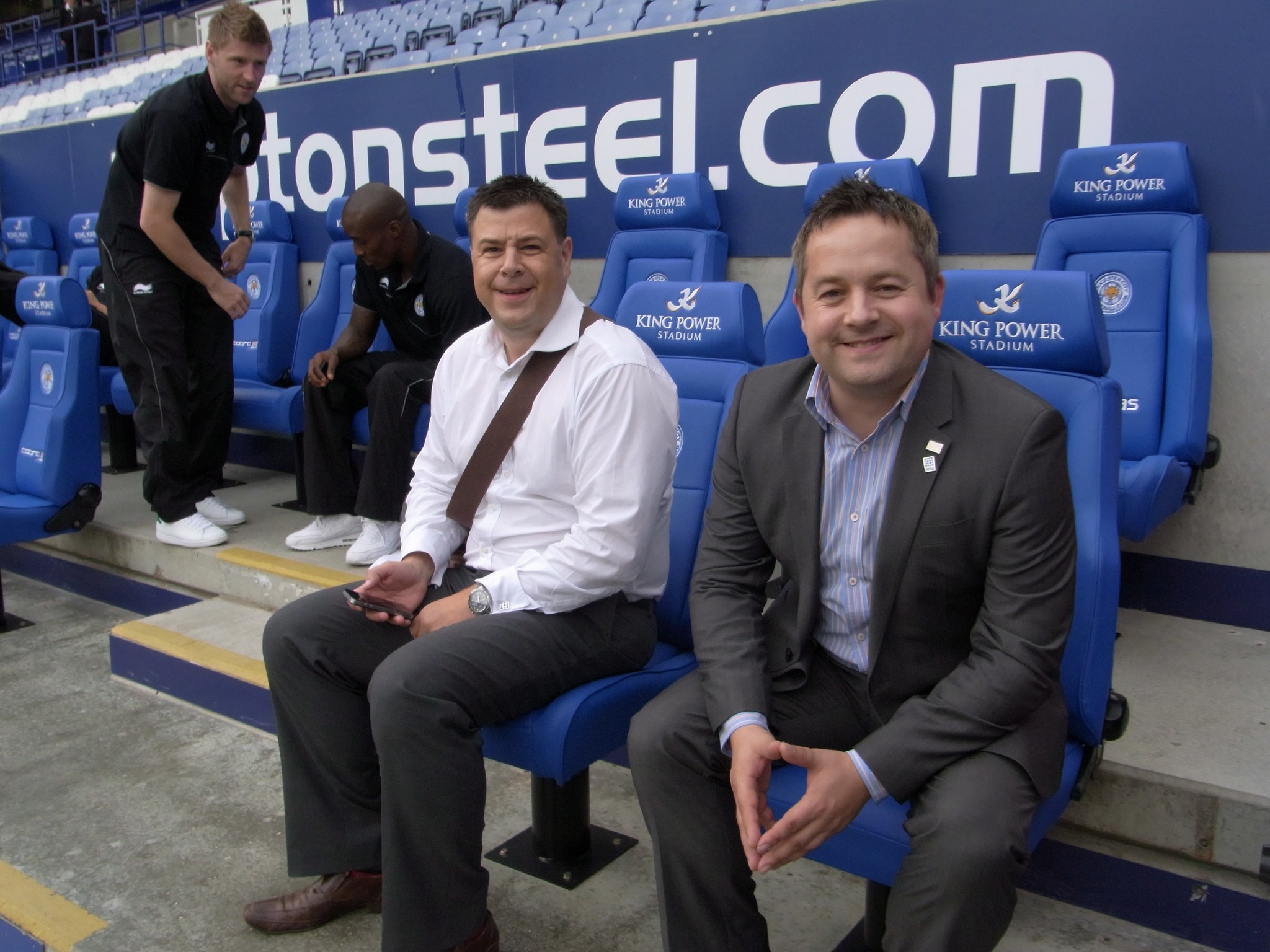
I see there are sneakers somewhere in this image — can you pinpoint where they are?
[156,512,228,548]
[194,497,247,525]
[286,514,363,551]
[345,518,402,566]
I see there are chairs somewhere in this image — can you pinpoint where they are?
[0,0,1209,951]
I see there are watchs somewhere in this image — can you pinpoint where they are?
[232,229,257,244]
[468,582,490,618]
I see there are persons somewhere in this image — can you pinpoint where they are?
[628,178,1078,952]
[241,174,680,952]
[284,183,488,566]
[95,6,272,546]
[70,0,108,71]
[59,0,77,72]
[0,261,118,367]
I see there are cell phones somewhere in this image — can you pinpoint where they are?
[342,588,416,621]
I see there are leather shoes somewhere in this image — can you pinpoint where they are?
[242,868,383,934]
[444,907,500,952]
